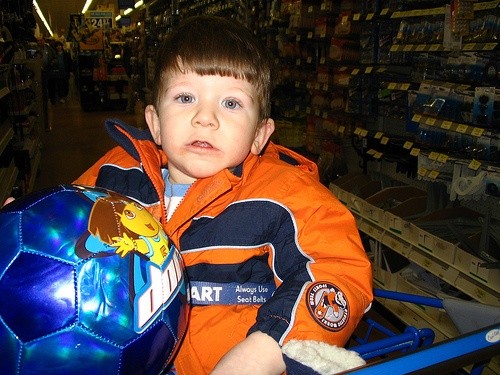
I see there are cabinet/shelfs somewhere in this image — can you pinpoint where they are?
[0,62,41,208]
[329,170,500,374]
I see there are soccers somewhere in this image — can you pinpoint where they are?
[0,182,191,375]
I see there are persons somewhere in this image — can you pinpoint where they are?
[53,40,74,105]
[0,12,373,375]
